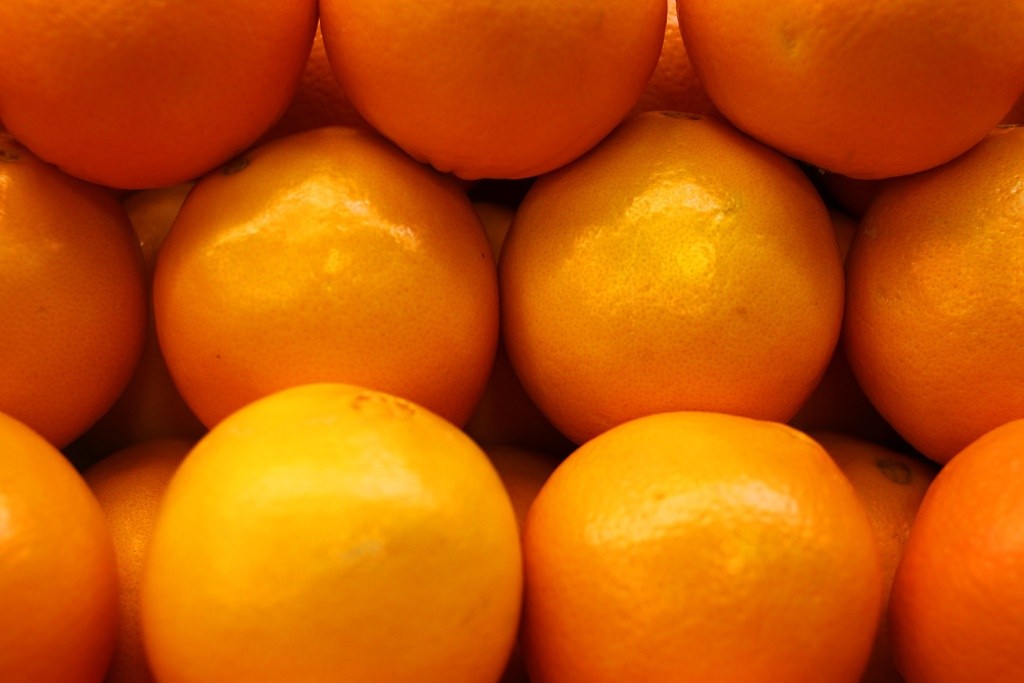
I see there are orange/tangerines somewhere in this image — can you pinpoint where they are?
[0,1,1023,683]
